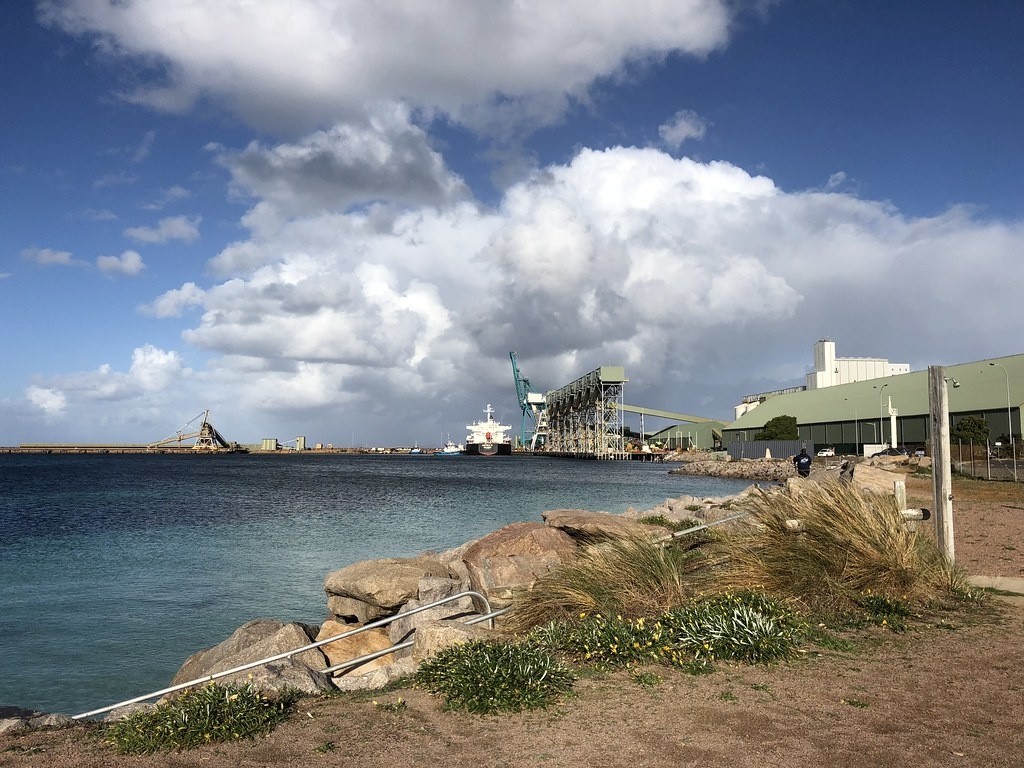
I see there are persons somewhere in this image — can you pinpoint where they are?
[793,449,812,478]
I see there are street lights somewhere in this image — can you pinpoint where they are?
[989,363,1012,445]
[873,384,888,444]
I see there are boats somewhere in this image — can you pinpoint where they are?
[464,403,513,456]
[409,440,423,454]
[434,432,463,456]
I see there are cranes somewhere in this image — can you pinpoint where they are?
[508,351,545,448]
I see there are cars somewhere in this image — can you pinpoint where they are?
[818,448,835,457]
[915,446,926,457]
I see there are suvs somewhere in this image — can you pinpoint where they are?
[872,447,913,459]
